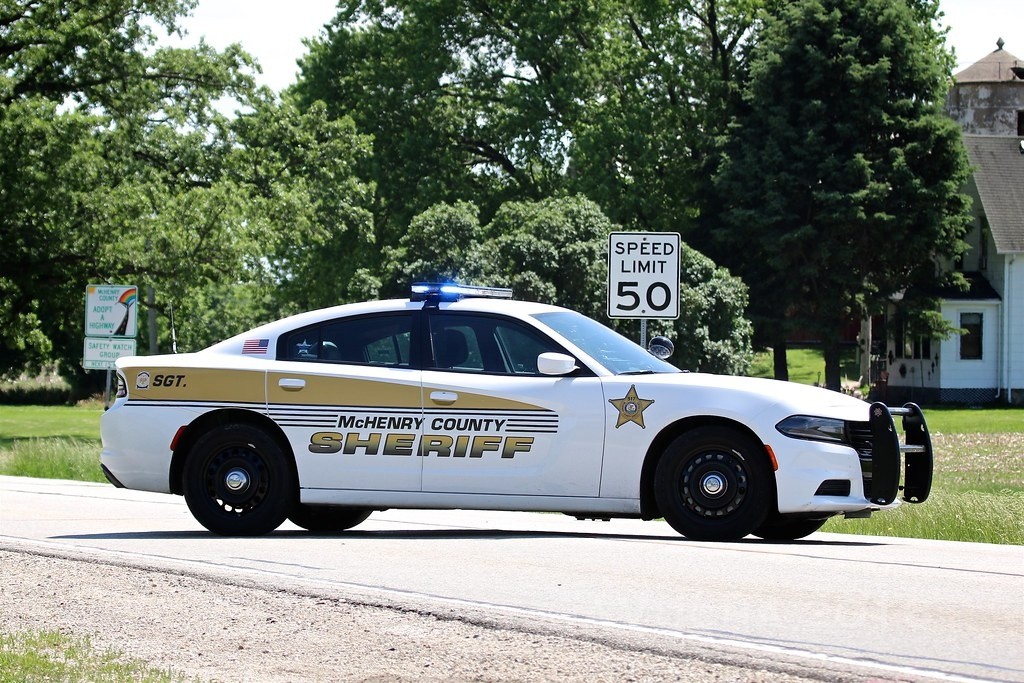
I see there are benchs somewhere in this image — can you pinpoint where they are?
[309,341,359,361]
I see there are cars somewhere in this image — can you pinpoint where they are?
[97,280,933,542]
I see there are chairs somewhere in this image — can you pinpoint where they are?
[433,329,471,368]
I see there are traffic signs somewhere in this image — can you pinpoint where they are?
[83,336,136,370]
[85,284,138,337]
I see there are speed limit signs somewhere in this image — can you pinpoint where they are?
[606,231,681,320]
